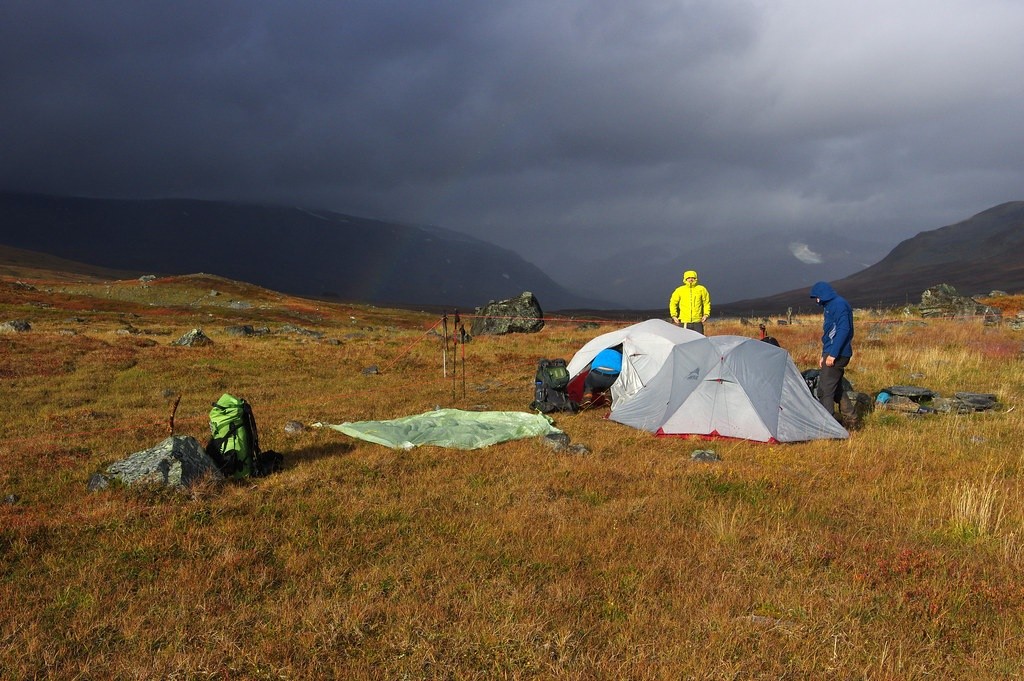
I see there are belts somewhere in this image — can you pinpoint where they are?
[592,369,619,377]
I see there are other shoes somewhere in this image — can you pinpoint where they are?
[579,399,592,407]
[605,397,613,406]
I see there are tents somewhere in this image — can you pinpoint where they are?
[471,291,544,336]
[565,317,851,444]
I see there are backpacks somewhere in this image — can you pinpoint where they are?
[532,357,569,412]
[208,392,261,480]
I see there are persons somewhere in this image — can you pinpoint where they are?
[578,349,622,409]
[810,281,860,424]
[669,271,710,335]
